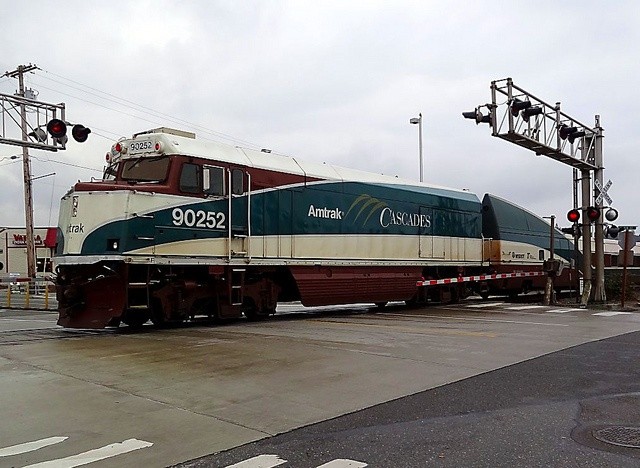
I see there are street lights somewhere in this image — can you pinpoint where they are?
[411,112,426,185]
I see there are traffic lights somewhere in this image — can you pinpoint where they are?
[512,98,531,117]
[55,135,68,143]
[606,209,618,220]
[522,107,542,122]
[72,125,90,143]
[568,210,579,221]
[557,124,577,139]
[588,207,600,221]
[47,119,66,138]
[569,131,585,144]
[31,128,47,141]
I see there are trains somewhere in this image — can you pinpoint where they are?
[57,128,640,328]
